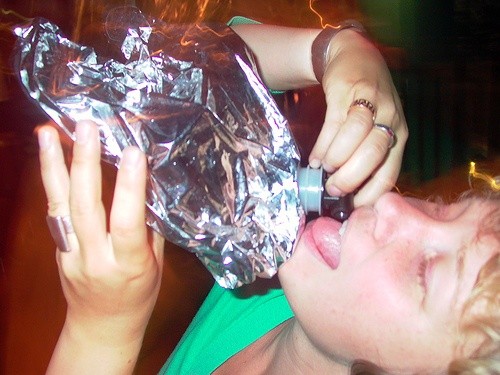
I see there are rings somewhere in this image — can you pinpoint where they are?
[347,98,375,122]
[372,123,397,148]
[45,216,74,252]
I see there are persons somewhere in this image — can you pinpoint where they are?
[33,16,500,375]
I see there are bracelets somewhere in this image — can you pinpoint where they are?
[312,20,368,84]
